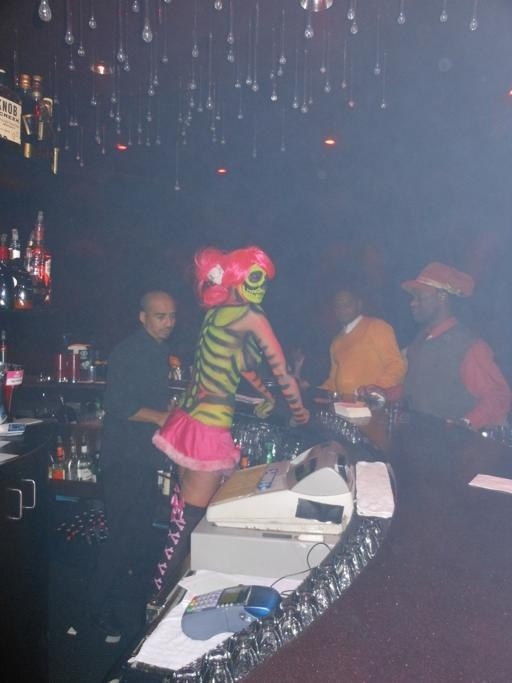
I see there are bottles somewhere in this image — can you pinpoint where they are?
[0,210,55,313]
[46,435,101,483]
[231,422,302,470]
[20,70,55,121]
[0,328,23,424]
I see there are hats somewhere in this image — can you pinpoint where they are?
[400,262,474,299]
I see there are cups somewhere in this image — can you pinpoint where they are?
[51,352,81,383]
[171,645,236,683]
[227,588,317,681]
[307,519,384,616]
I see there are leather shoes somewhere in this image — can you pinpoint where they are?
[86,602,123,636]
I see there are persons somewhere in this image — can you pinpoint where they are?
[285,340,329,391]
[291,282,405,397]
[142,242,309,622]
[358,261,510,431]
[85,289,177,612]
